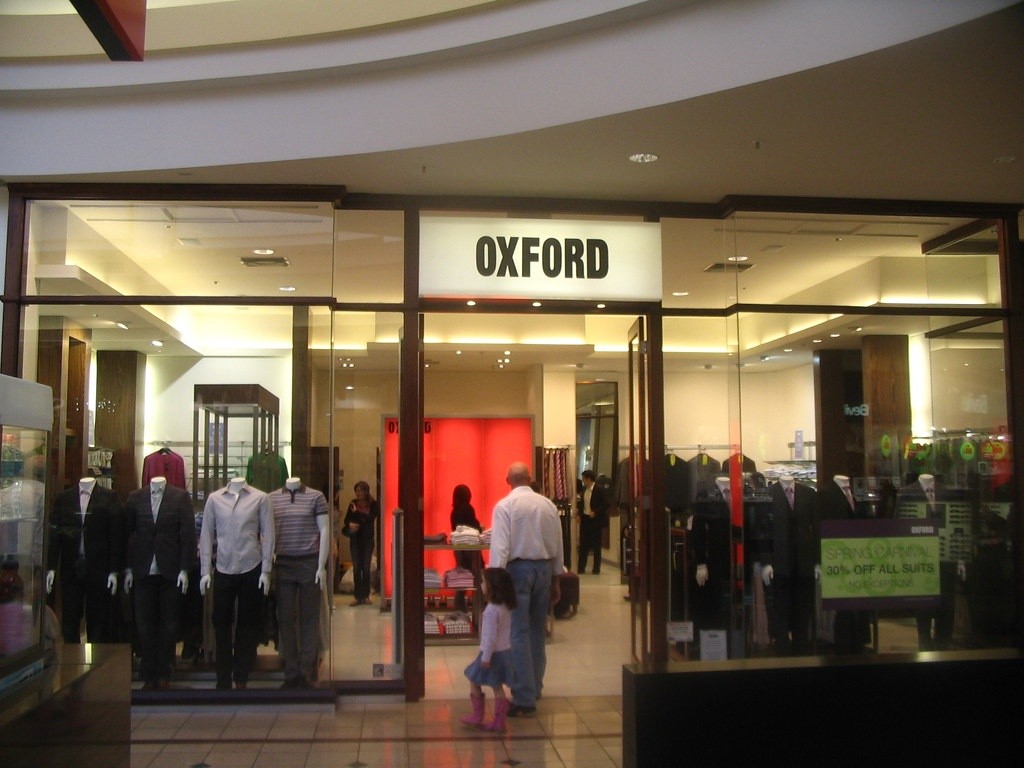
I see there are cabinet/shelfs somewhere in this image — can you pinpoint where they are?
[422,544,555,646]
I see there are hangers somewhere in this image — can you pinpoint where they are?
[262,443,275,455]
[699,448,709,458]
[666,447,676,459]
[159,442,173,454]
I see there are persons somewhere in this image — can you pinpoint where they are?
[45,477,122,644]
[691,476,757,661]
[199,476,275,689]
[488,462,564,716]
[451,484,484,612]
[762,474,823,658]
[333,483,341,609]
[817,476,873,651]
[893,474,967,651]
[460,567,518,732]
[343,481,380,606]
[268,477,330,688]
[578,470,609,574]
[0,455,49,597]
[123,477,197,689]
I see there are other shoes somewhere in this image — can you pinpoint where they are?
[236,681,246,690]
[577,569,585,574]
[349,597,373,606]
[141,677,169,691]
[508,704,536,716]
[592,569,600,575]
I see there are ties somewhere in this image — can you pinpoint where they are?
[723,489,730,512]
[842,487,854,511]
[786,488,794,510]
[927,488,935,511]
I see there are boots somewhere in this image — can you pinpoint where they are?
[481,698,510,731]
[458,693,485,726]
